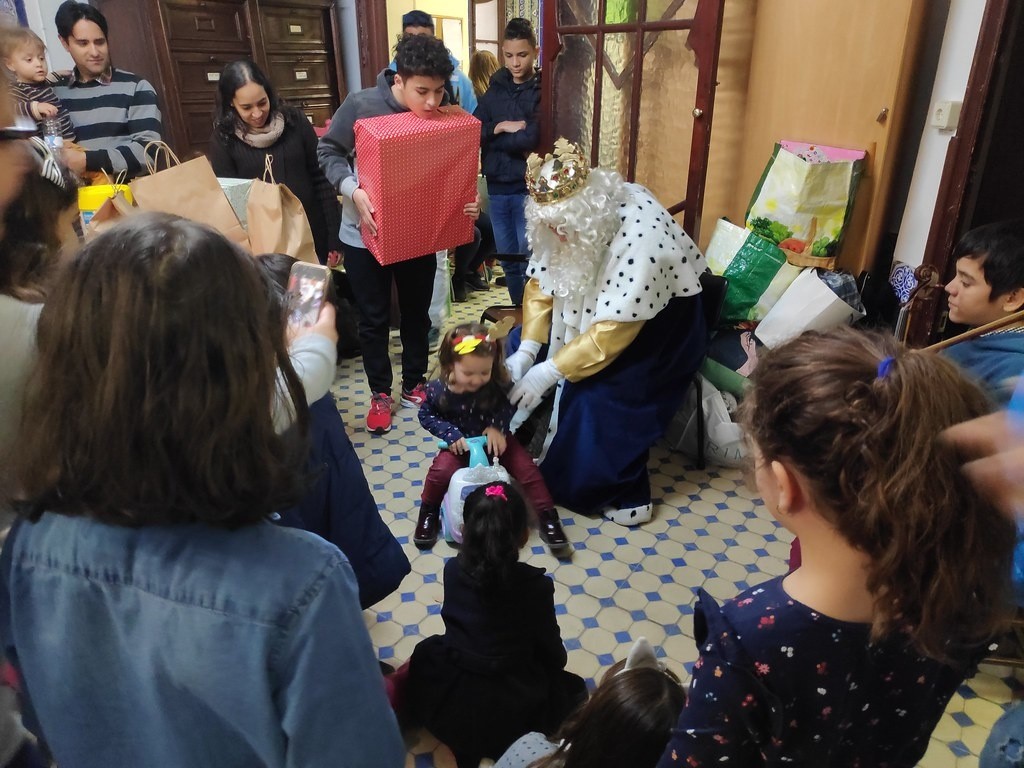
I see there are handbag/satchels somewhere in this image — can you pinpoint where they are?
[265,390,411,610]
[80,139,320,270]
[667,142,956,468]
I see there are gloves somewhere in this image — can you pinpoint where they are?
[506,356,564,411]
[501,350,534,384]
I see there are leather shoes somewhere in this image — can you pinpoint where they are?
[538,506,571,547]
[414,504,439,544]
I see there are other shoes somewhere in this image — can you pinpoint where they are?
[496,276,508,286]
[426,326,440,351]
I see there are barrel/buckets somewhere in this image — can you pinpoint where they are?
[78,184,133,242]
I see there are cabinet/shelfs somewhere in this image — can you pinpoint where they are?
[86,0,347,178]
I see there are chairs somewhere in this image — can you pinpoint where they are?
[480,242,730,472]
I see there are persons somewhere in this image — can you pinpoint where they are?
[384,482,590,763]
[491,223,1024,768]
[448,16,542,304]
[414,319,570,548]
[390,11,477,355]
[0,0,341,528]
[316,32,457,434]
[504,138,710,525]
[1,212,404,768]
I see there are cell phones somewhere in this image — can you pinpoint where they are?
[283,262,331,343]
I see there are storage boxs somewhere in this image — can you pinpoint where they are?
[352,104,481,268]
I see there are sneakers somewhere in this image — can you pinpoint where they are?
[465,272,491,291]
[365,392,396,431]
[450,276,467,302]
[399,380,429,407]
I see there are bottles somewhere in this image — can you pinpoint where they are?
[42,116,64,172]
